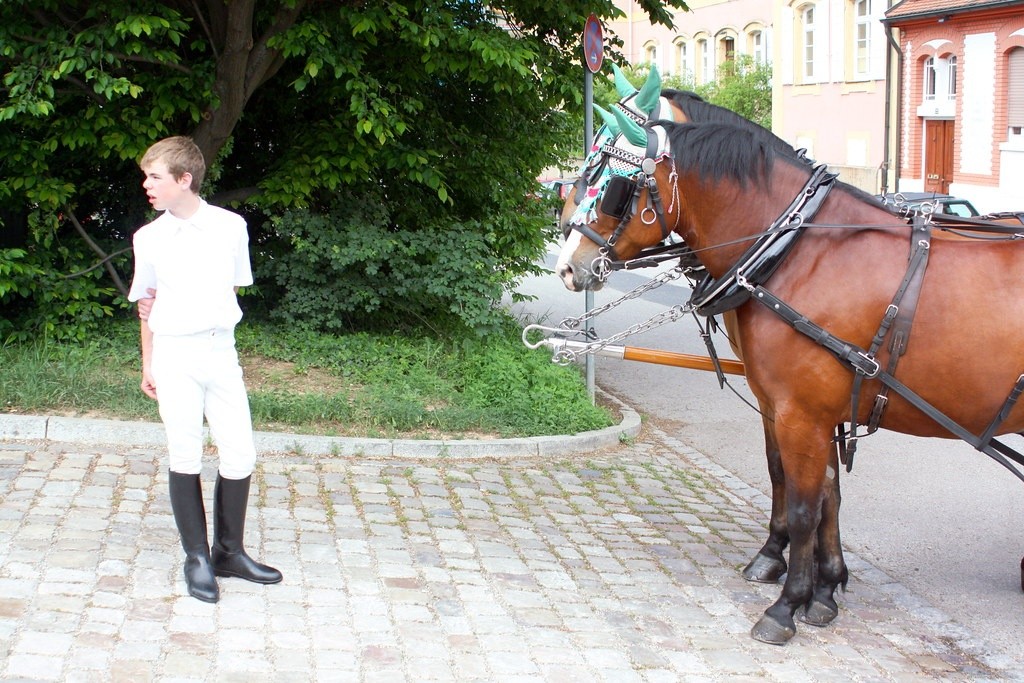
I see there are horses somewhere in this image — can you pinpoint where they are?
[553,62,1024,646]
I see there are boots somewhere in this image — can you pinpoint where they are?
[210,470,282,583]
[169,469,218,603]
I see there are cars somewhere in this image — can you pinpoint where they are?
[540,180,576,229]
[873,192,980,218]
[517,178,563,213]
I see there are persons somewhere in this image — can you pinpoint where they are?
[127,136,284,603]
